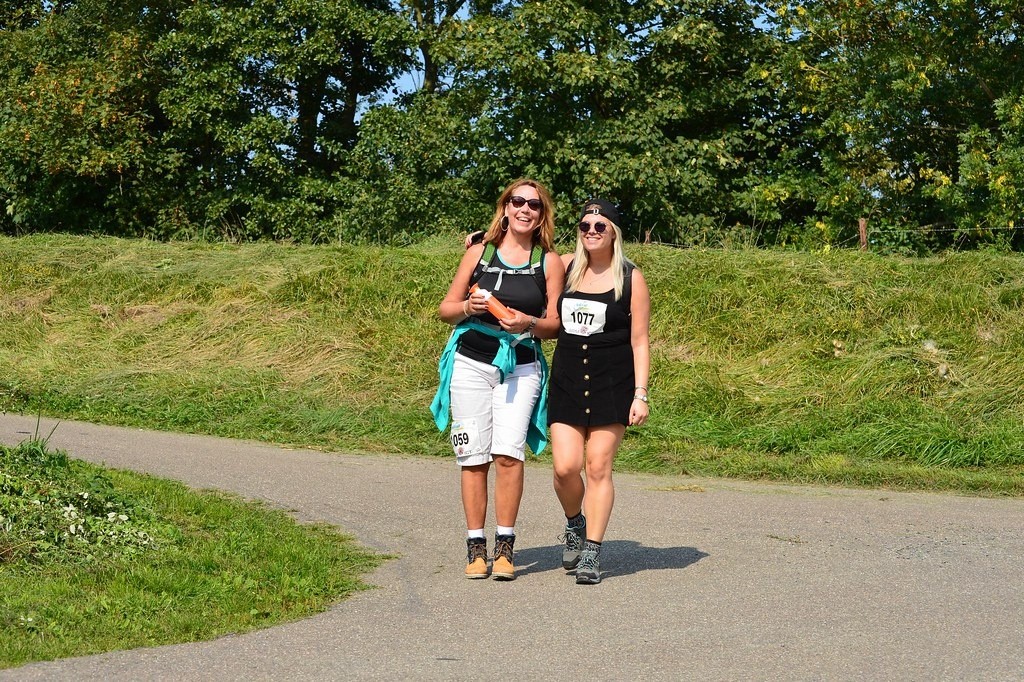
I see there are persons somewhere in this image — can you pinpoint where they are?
[437,179,650,585]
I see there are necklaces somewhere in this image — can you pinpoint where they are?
[587,262,612,285]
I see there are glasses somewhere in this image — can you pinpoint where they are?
[510,196,542,210]
[579,222,605,232]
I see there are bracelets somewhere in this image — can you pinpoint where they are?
[463,300,471,317]
[526,316,537,331]
[634,386,648,394]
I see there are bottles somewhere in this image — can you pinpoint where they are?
[469,283,514,321]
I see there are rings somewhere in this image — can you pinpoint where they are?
[512,327,515,332]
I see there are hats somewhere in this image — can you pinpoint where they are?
[580,199,619,227]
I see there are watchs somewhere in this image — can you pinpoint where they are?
[634,395,649,403]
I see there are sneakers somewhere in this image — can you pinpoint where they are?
[491,532,515,579]
[465,536,488,578]
[557,515,586,570]
[576,550,600,583]
